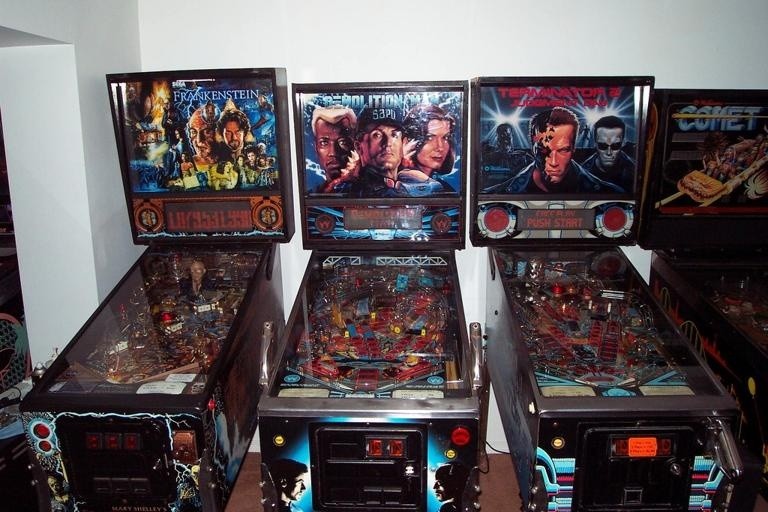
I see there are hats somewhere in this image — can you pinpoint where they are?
[357,99,408,136]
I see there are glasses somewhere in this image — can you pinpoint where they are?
[596,141,622,150]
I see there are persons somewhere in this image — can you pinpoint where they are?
[480,123,534,189]
[334,98,445,198]
[48,451,73,512]
[124,86,275,192]
[270,458,308,512]
[307,103,357,194]
[701,125,768,185]
[580,116,634,194]
[433,460,470,512]
[397,104,457,192]
[480,106,624,194]
